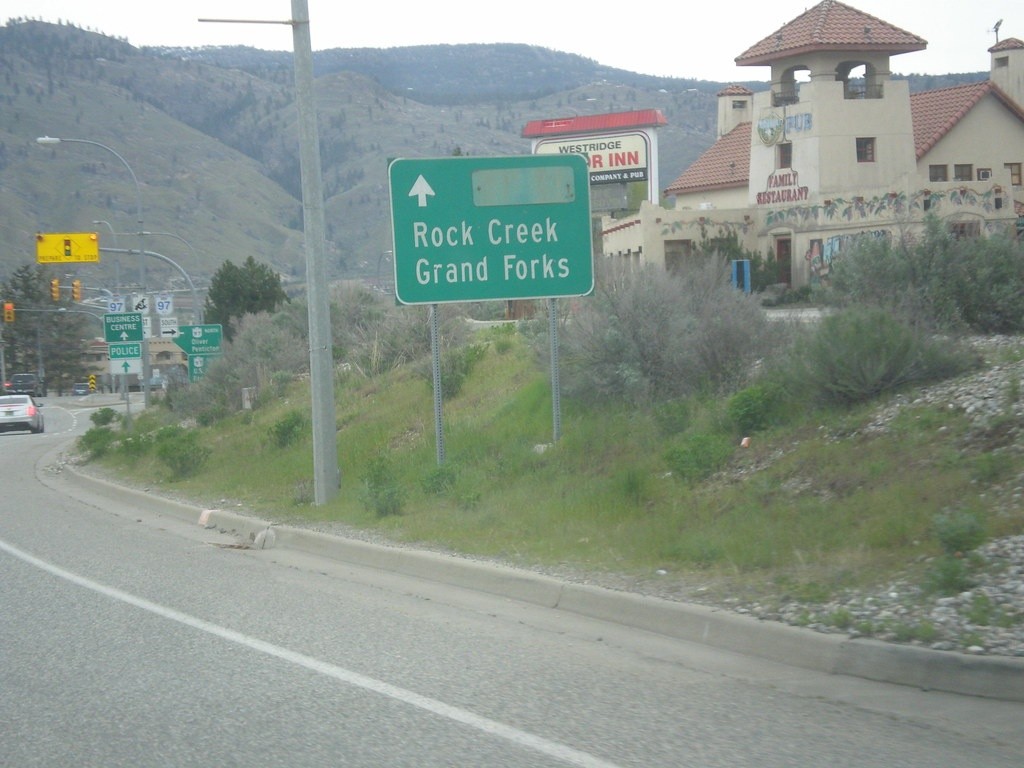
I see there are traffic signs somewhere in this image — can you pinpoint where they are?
[388,152,595,306]
[108,343,142,375]
[187,354,218,383]
[160,317,180,338]
[103,311,144,343]
[171,324,224,355]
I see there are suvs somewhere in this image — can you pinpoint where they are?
[5,373,38,398]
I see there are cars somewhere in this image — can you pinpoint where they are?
[0,394,45,434]
[72,383,90,395]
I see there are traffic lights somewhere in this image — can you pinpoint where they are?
[72,280,80,302]
[4,302,14,322]
[50,279,59,300]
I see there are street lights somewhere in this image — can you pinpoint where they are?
[36,137,148,297]
[92,220,120,297]
[37,307,66,397]
